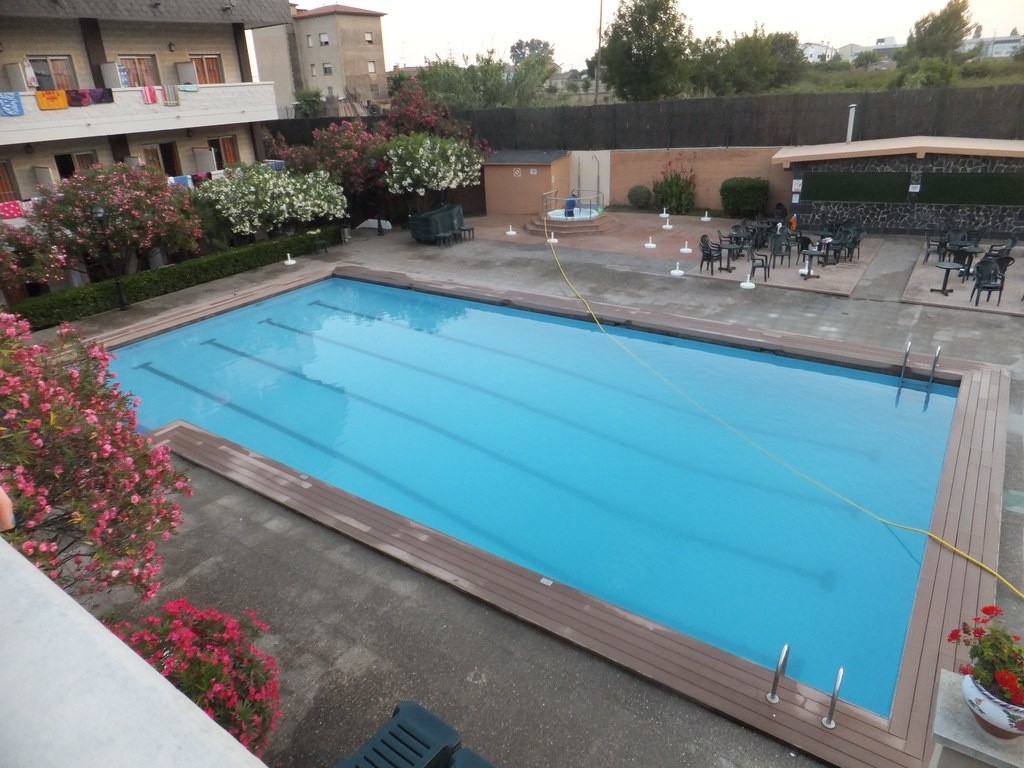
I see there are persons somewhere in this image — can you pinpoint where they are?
[773,202,786,228]
[565,194,577,218]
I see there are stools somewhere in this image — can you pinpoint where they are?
[434,225,475,246]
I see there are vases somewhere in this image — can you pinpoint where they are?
[961,663,1024,739]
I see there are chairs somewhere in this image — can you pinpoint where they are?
[699,202,867,281]
[922,228,1018,306]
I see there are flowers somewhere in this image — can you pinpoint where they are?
[947,605,1024,708]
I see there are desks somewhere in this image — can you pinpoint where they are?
[927,241,985,296]
[719,219,839,279]
